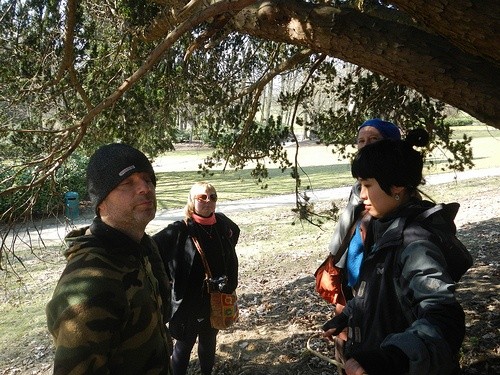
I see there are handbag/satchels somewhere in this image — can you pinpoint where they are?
[316,254,346,307]
[210,290,239,329]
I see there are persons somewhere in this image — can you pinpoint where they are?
[321,139,473,375]
[328,120,402,341]
[46,143,176,375]
[152,182,240,375]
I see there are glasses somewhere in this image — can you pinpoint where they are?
[193,195,218,202]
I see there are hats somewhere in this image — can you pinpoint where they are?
[358,119,400,141]
[87,142,156,217]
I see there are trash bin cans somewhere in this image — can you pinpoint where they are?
[64,191,79,219]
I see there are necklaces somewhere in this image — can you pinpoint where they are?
[199,224,212,238]
